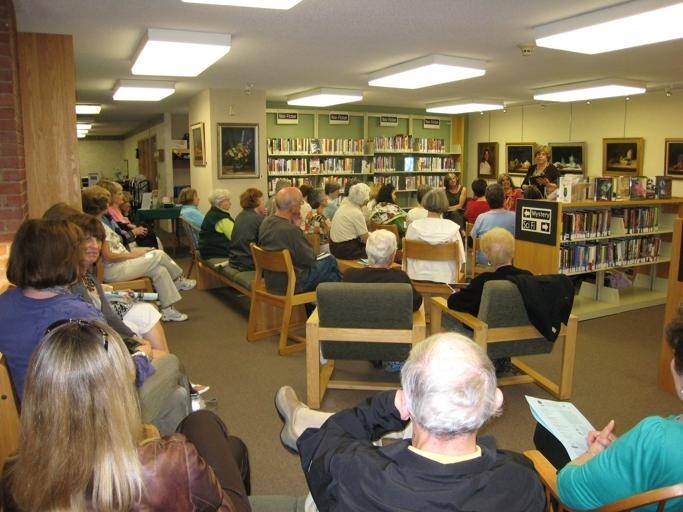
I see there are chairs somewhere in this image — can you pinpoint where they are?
[0,356,160,459]
[92,258,156,307]
[519,445,682,511]
[180,217,578,409]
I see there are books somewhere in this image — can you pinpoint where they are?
[264,135,456,196]
[555,172,672,271]
[524,396,596,461]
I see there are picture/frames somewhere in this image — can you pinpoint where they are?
[545,141,588,177]
[504,141,536,178]
[188,121,206,166]
[661,136,683,182]
[214,118,259,180]
[600,135,644,180]
[476,140,499,181]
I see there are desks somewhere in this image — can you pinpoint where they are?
[137,205,181,257]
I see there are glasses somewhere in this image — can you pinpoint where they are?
[44,318,108,352]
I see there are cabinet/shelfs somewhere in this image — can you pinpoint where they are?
[266,144,460,212]
[657,213,682,398]
[513,195,682,322]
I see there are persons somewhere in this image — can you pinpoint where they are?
[271,330,550,512]
[0,318,254,512]
[533,304,682,512]
[553,156,565,170]
[0,174,209,397]
[478,148,492,176]
[520,145,558,199]
[178,175,534,372]
[623,147,632,166]
[564,150,580,169]
[0,221,194,440]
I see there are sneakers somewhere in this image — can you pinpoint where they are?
[275,385,310,452]
[161,304,189,322]
[174,273,197,291]
[189,382,209,395]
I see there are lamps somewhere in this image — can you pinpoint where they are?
[111,78,176,102]
[532,77,646,103]
[425,97,505,114]
[286,88,362,107]
[532,2,682,55]
[128,27,233,78]
[368,54,487,90]
